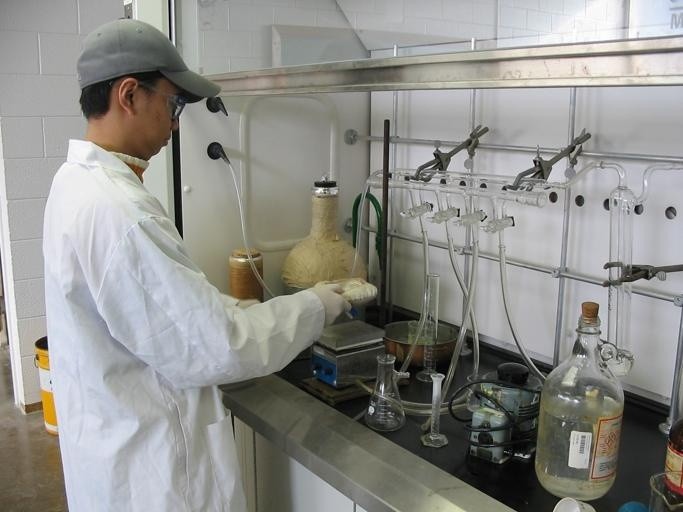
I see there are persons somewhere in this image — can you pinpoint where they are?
[41,17,349,512]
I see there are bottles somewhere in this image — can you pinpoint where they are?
[662,417,683,498]
[421,371,449,448]
[534,302,627,503]
[414,273,442,384]
[364,353,407,433]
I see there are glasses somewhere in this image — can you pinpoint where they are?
[106,76,188,121]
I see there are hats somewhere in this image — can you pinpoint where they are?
[73,16,222,105]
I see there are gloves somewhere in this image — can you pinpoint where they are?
[311,277,351,326]
